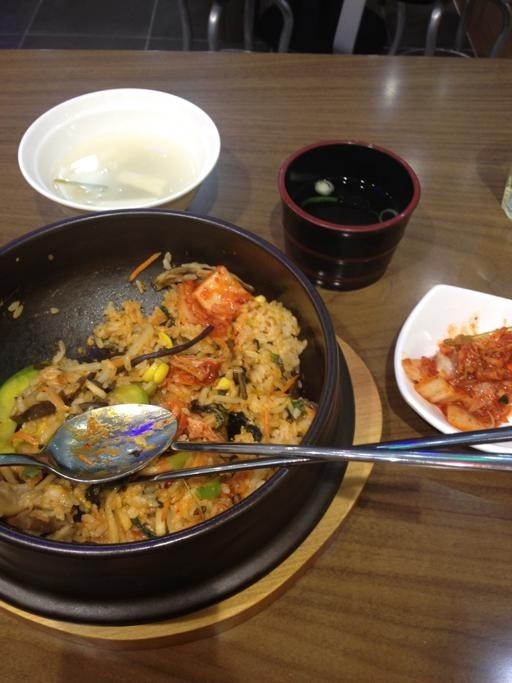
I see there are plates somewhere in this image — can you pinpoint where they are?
[395,282,512,458]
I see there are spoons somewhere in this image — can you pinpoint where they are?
[2,403,178,483]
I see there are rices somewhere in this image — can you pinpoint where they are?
[0,252,309,544]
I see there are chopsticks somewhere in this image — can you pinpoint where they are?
[134,426,510,481]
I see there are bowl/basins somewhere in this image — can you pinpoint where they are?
[17,90,222,212]
[0,213,346,603]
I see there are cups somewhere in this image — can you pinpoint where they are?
[279,139,419,288]
